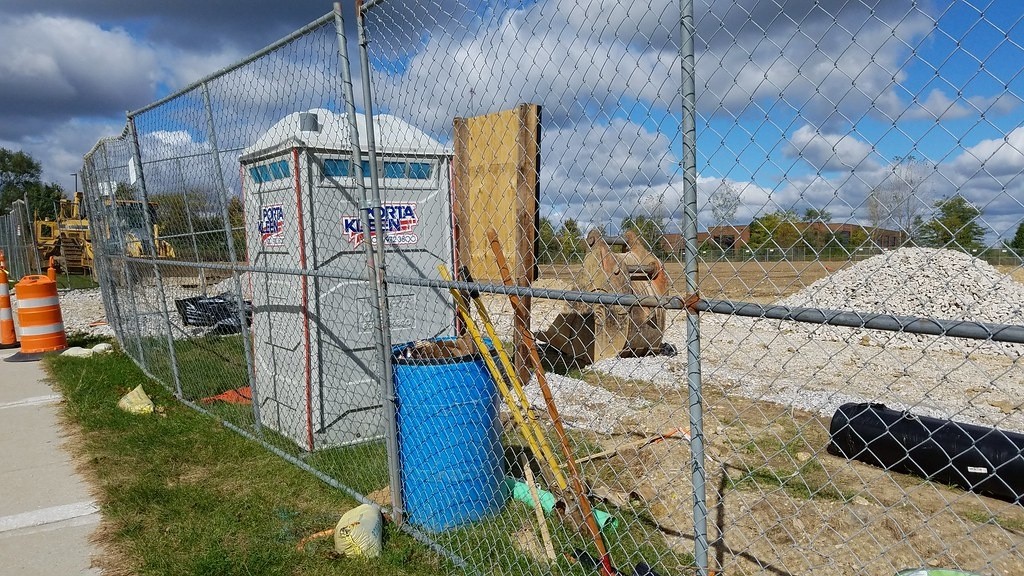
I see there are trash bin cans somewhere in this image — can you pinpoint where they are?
[393,333,511,532]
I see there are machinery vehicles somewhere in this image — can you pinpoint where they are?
[26,191,177,284]
